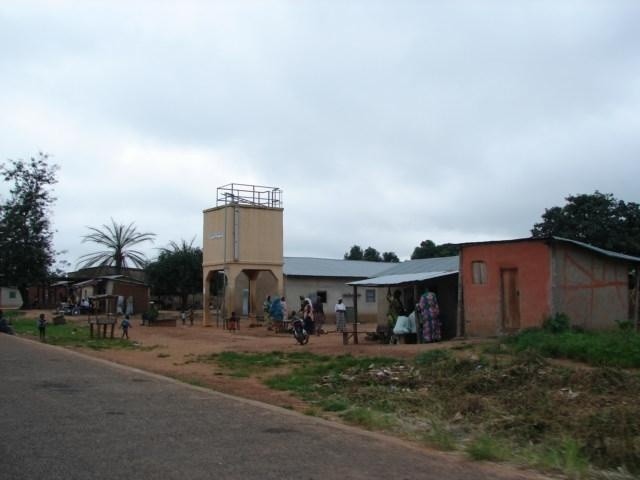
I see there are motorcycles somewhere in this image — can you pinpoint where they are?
[287,308,310,346]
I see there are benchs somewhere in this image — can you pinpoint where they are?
[148,318,176,327]
[396,333,416,344]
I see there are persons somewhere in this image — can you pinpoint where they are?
[225,311,238,333]
[263,286,443,343]
[118,314,133,341]
[81,299,89,307]
[140,301,159,328]
[180,310,187,324]
[37,313,48,339]
[186,308,194,326]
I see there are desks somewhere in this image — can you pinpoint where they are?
[327,332,379,344]
[274,320,292,334]
[223,317,240,330]
